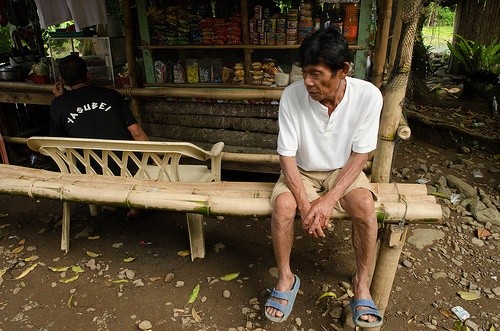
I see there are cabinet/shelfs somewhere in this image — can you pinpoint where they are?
[136,0,376,85]
[48,32,127,86]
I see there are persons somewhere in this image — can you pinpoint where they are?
[48,55,150,218]
[264,27,383,328]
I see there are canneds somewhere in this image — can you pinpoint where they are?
[249,2,320,45]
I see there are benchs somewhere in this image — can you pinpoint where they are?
[28,134,225,261]
[0,163,443,331]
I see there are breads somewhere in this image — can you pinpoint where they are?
[233,61,279,86]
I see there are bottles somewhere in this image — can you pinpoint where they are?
[174,59,222,83]
[344,18,358,42]
[313,14,320,31]
[323,13,331,30]
[331,17,342,35]
[30,152,38,166]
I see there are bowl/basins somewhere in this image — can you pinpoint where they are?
[116,77,129,86]
[29,72,50,85]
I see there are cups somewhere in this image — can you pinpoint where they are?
[274,73,289,86]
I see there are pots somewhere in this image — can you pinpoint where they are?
[0,66,26,82]
[9,55,33,66]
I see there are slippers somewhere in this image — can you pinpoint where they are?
[264,274,300,323]
[350,297,384,328]
